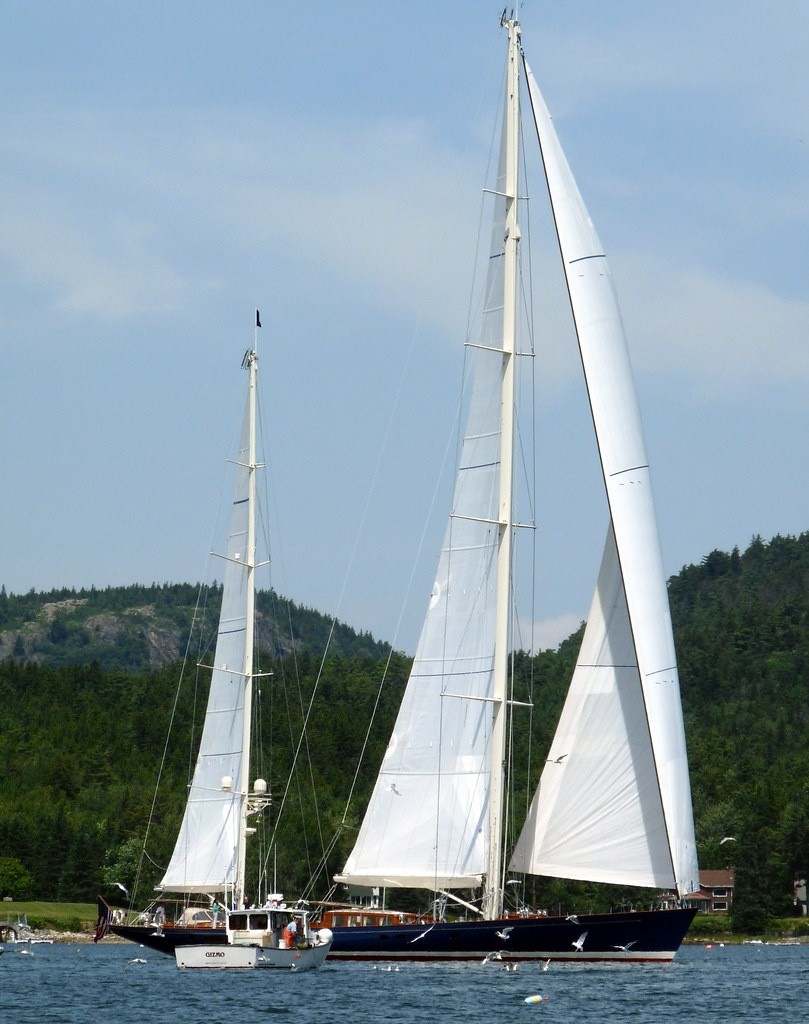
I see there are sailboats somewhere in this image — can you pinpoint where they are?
[106,306,313,957]
[290,0,703,962]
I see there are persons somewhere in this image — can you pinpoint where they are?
[245,894,249,909]
[284,916,304,948]
[504,907,548,919]
[212,898,220,929]
[156,903,164,921]
[266,898,280,909]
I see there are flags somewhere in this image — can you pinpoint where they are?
[255,309,262,327]
[94,894,113,943]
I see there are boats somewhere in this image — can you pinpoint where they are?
[174,843,334,970]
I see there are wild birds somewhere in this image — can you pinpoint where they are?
[18,882,147,966]
[719,837,738,845]
[373,913,636,972]
